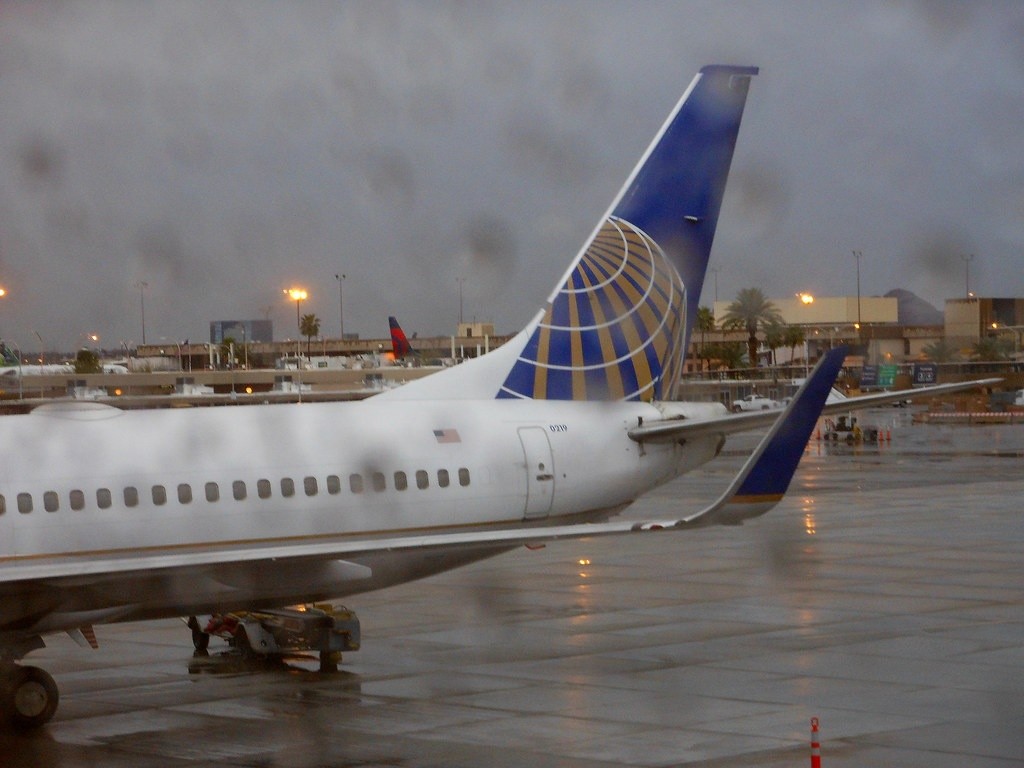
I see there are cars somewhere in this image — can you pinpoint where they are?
[732,393,779,414]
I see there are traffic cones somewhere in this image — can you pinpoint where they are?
[878,426,884,441]
[886,425,891,441]
[815,426,821,439]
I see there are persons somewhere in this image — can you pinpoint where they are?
[186,616,217,675]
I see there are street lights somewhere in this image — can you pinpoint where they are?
[291,289,308,331]
[852,249,863,338]
[136,281,148,346]
[335,273,345,340]
[962,253,975,299]
[456,276,466,325]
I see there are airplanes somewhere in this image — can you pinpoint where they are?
[0,67,848,730]
